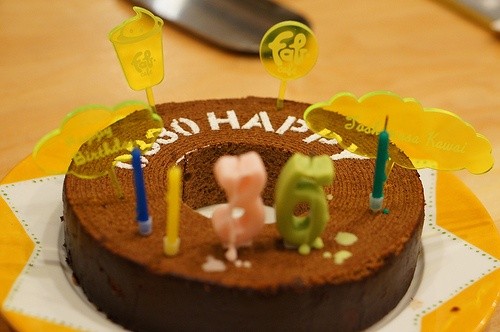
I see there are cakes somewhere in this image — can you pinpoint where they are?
[62,96,424,332]
